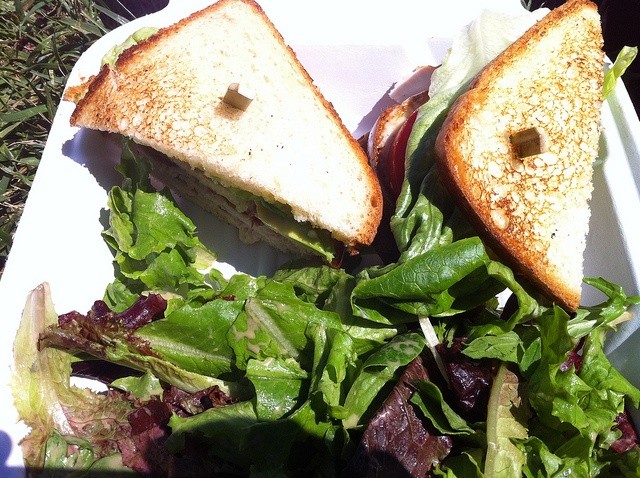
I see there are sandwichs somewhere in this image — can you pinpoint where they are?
[366,0,605,319]
[64,0,384,265]
